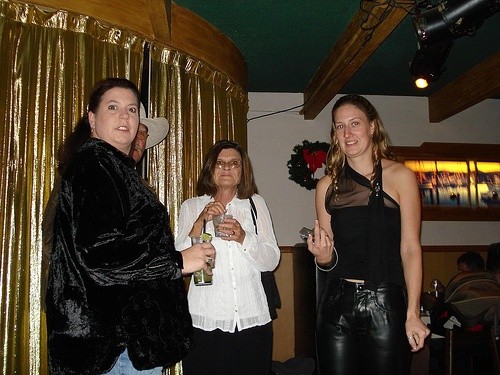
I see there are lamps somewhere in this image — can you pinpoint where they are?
[409,0,500,91]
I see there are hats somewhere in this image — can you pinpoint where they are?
[139,101,169,149]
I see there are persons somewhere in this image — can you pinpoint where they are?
[174,139,280,375]
[43,77,216,375]
[307,93,500,375]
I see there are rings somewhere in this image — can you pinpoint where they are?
[206,257,213,266]
[207,207,210,212]
[232,230,235,235]
[326,235,329,237]
[408,337,413,339]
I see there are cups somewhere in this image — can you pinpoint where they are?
[430,280,442,302]
[212,215,234,237]
[192,236,213,286]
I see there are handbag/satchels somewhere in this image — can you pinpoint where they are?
[261,272,281,319]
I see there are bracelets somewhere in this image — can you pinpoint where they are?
[315,247,339,272]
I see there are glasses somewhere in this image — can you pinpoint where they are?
[137,130,149,140]
[214,159,243,170]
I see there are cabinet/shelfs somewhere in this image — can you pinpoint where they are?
[171,246,315,375]
